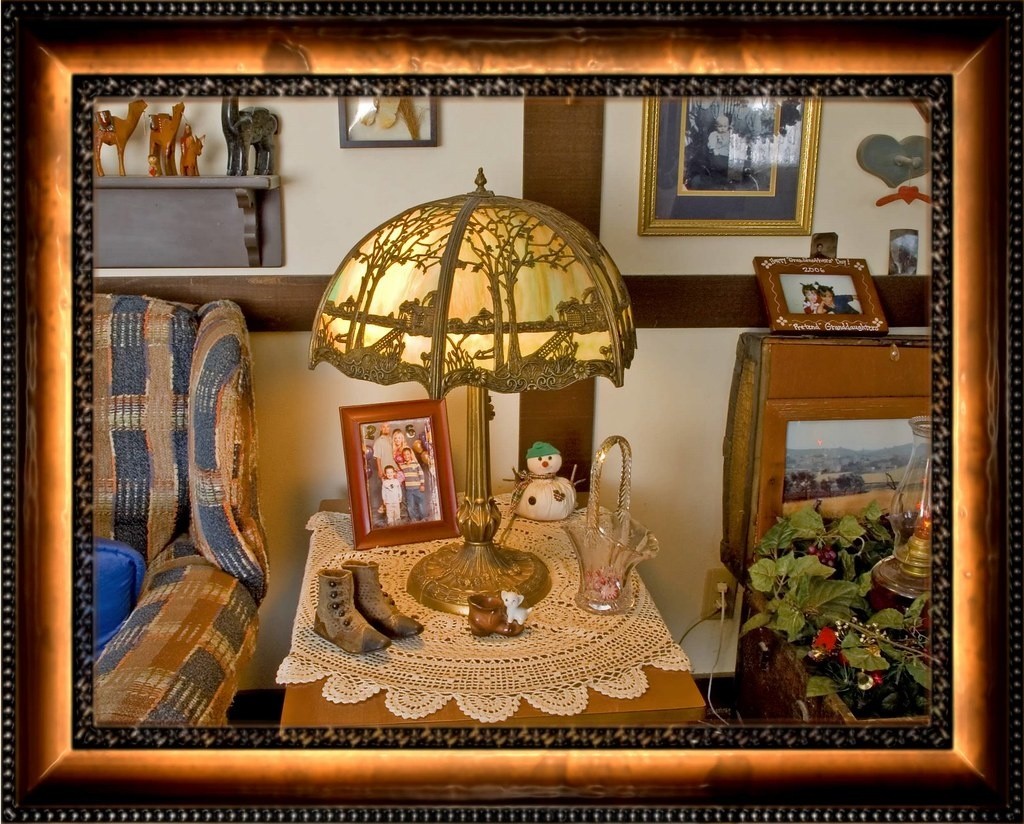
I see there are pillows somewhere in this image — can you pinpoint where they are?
[93,536,147,661]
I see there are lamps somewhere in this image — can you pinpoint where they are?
[307,165,638,613]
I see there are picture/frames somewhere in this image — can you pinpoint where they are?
[339,398,462,551]
[755,256,890,339]
[337,94,438,149]
[0,0,1024,824]
[638,99,822,235]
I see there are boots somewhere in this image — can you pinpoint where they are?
[343,560,424,638]
[314,569,392,655]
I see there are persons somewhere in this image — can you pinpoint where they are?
[811,243,830,260]
[180,122,203,176]
[148,155,159,177]
[800,282,860,315]
[689,96,762,185]
[371,421,429,527]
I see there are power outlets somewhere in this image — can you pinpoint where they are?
[701,566,737,621]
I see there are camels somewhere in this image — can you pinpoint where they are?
[93,99,185,176]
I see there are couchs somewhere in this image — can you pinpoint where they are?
[91,291,268,730]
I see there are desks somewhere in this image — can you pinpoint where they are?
[280,491,708,729]
